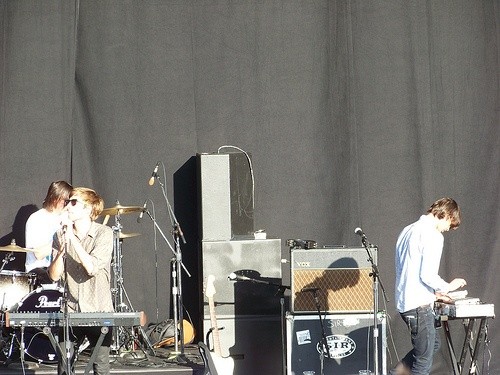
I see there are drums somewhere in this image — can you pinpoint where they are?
[1,269,37,312]
[14,287,80,363]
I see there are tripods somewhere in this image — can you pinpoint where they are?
[111,172,203,371]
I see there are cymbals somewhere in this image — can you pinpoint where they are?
[119,232,142,239]
[0,245,35,252]
[101,205,144,214]
[286,238,317,249]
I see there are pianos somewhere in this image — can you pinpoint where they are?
[434,289,496,375]
[0,310,146,375]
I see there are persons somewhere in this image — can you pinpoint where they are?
[24,181,74,289]
[389,196,467,375]
[50,188,114,375]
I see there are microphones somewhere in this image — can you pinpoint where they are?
[301,288,319,292]
[149,162,160,185]
[355,227,366,239]
[229,273,251,281]
[63,225,67,233]
[137,201,148,223]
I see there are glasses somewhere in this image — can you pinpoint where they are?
[64,199,86,207]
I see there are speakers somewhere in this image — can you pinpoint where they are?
[198,151,286,375]
[290,245,378,314]
